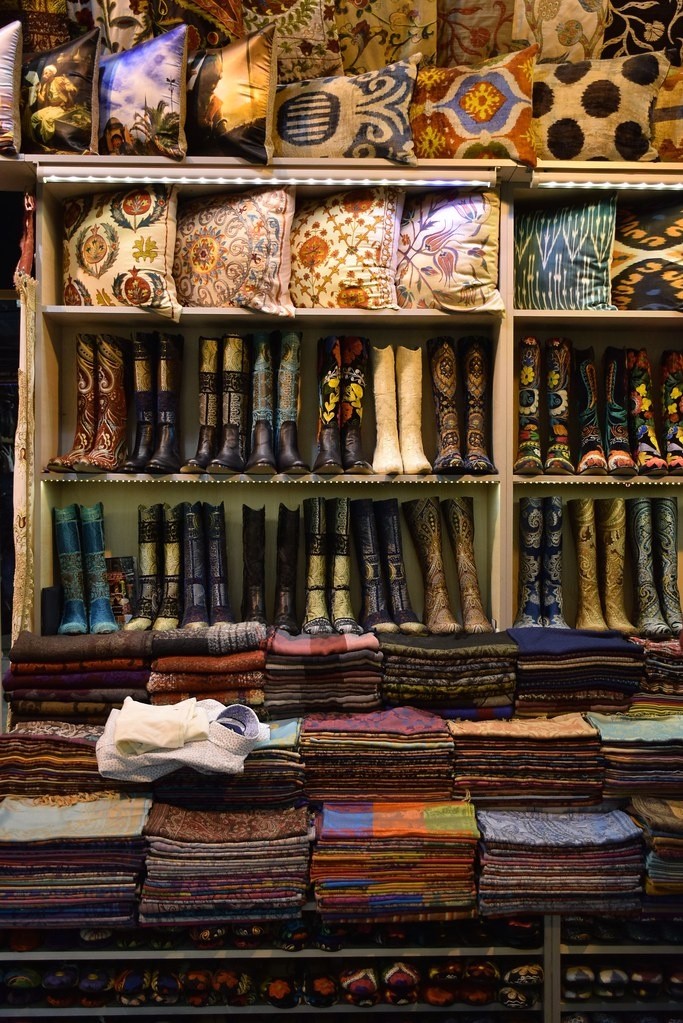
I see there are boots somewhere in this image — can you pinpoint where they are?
[513,496,571,630]
[53,502,119,635]
[241,504,269,626]
[626,497,683,638]
[274,503,300,635]
[47,333,132,473]
[117,330,186,474]
[440,496,495,634]
[180,333,252,474]
[303,497,364,635]
[244,330,312,467]
[457,335,498,475]
[313,336,374,474]
[569,343,640,475]
[372,343,433,475]
[623,347,683,475]
[181,502,236,629]
[566,498,640,636]
[425,335,465,473]
[123,504,184,631]
[400,496,463,634]
[513,335,571,475]
[350,498,429,636]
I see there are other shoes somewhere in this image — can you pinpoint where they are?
[0,956,683,1023]
[0,918,683,952]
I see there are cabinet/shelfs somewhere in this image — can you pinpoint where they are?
[19,156,508,642]
[508,163,683,627]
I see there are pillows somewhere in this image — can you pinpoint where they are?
[64,191,506,325]
[0,20,683,167]
[515,193,683,314]
